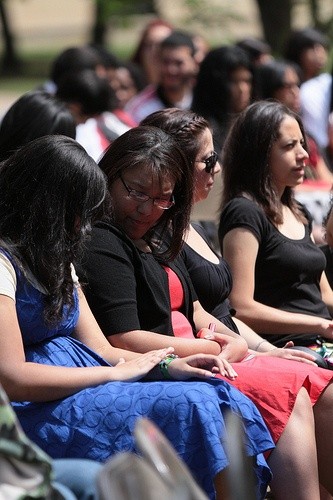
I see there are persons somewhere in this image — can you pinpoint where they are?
[0,92,77,164]
[216,97,333,369]
[139,107,328,369]
[33,17,333,290]
[0,136,280,500]
[75,125,333,500]
[0,384,108,499]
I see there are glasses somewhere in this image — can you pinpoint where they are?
[194,150,218,172]
[118,174,176,209]
